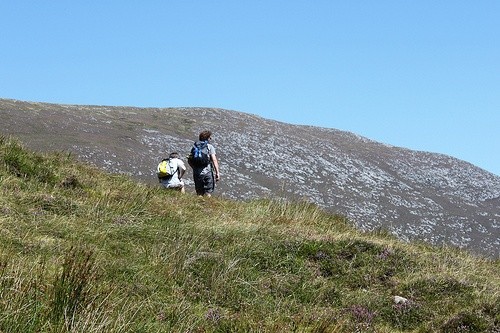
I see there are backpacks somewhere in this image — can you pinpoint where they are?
[192,141,210,164]
[157,158,174,178]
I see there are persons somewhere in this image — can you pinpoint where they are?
[158,153,186,196]
[188,129,220,199]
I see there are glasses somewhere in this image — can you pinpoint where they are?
[209,137,211,139]
